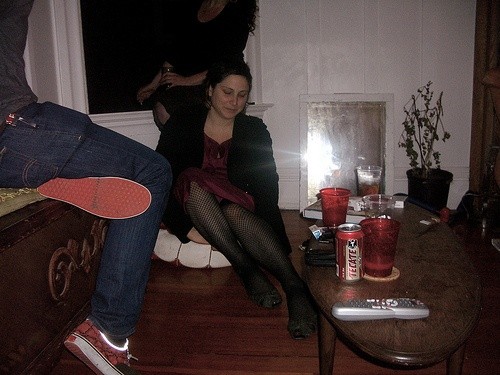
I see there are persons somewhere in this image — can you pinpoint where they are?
[0,0,172,375]
[170,54,320,341]
[137,0,259,153]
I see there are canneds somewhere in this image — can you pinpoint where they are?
[335,223,364,281]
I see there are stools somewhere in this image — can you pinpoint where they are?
[0,187,111,375]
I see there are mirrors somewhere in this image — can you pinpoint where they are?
[300,92,395,220]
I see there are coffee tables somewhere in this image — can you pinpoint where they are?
[306,194,480,375]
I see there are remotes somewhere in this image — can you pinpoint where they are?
[332,297,430,322]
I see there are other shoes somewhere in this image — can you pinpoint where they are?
[287,312,316,340]
[242,282,282,308]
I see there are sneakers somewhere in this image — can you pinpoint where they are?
[37,176,152,219]
[64,319,139,375]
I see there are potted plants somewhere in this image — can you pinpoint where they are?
[398,81,453,212]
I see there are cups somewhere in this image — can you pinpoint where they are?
[363,196,394,220]
[356,165,381,197]
[318,187,350,232]
[160,66,175,89]
[362,220,398,278]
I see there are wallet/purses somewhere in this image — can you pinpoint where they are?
[304,234,337,266]
